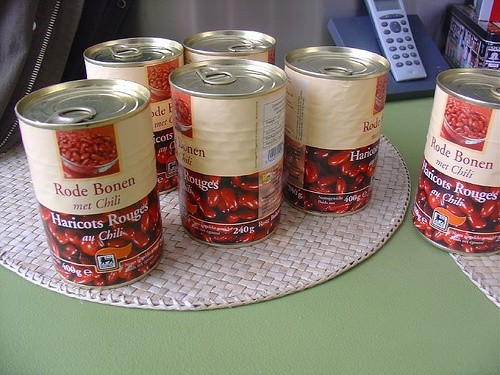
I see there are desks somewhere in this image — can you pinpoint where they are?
[0,98,500,375]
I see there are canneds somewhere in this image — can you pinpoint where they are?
[410,68,500,256]
[168,59,287,248]
[283,46,390,217]
[15,80,163,288]
[181,30,276,66]
[83,37,185,194]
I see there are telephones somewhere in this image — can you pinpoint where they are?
[327,0,452,101]
